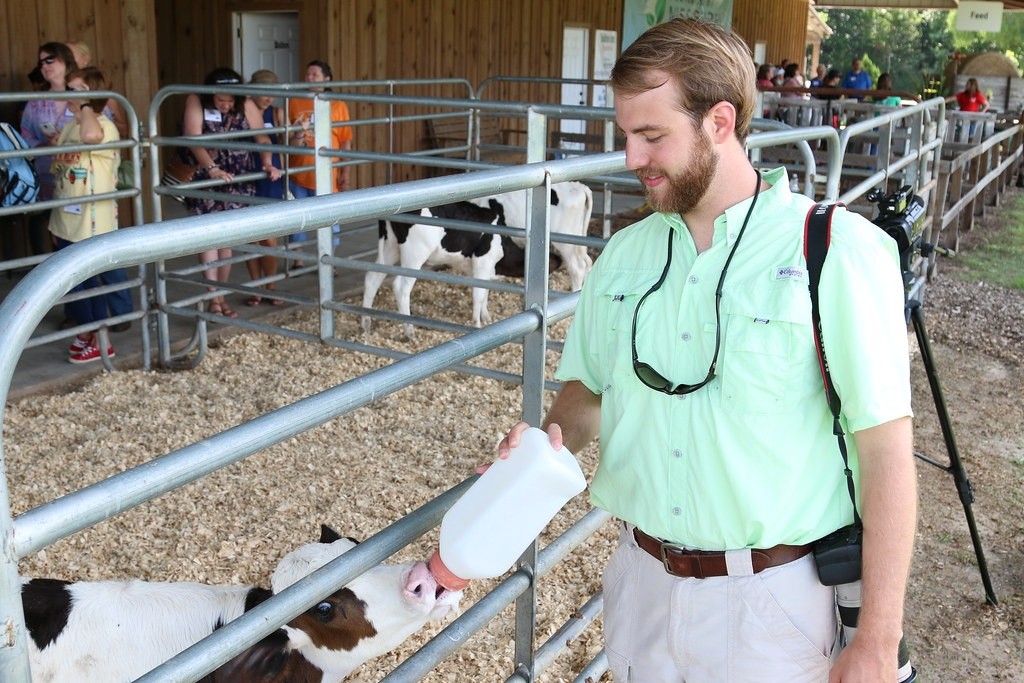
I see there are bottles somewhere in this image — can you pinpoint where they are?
[790,174,800,194]
[427,427,587,592]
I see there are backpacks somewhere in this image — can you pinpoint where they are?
[0,123,41,207]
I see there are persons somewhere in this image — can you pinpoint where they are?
[944,77,990,182]
[475,17,923,683]
[286,59,353,277]
[164,67,283,326]
[753,57,902,173]
[244,69,309,313]
[20,41,133,364]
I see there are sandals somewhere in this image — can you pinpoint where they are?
[207,303,238,323]
[245,288,283,306]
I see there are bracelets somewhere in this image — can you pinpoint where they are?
[80,102,92,110]
[293,133,306,140]
[206,164,218,173]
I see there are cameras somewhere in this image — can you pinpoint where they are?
[815,522,918,683]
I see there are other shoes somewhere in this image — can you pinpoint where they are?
[108,322,132,333]
[60,320,84,330]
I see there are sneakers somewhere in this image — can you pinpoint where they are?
[68,334,115,363]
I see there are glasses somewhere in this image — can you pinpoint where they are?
[65,85,75,91]
[38,55,57,67]
[631,284,721,394]
[216,75,239,83]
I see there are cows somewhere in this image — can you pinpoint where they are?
[358,180,595,349]
[17,524,462,683]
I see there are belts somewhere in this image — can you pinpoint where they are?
[624,522,812,579]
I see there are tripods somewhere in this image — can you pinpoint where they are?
[876,219,999,607]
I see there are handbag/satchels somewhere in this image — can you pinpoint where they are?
[161,158,195,203]
[116,160,134,190]
